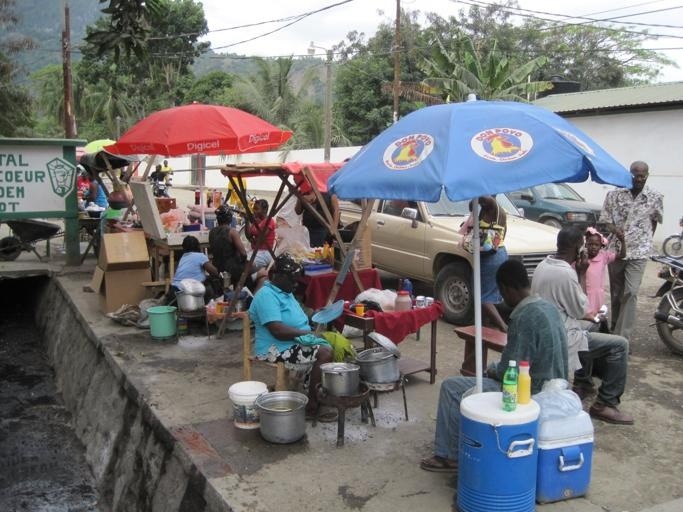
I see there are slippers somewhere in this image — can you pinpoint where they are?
[304,409,338,423]
[421,455,457,473]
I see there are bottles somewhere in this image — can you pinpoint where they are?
[501,360,531,412]
[194,186,226,209]
[395,273,414,313]
[215,301,231,314]
[314,239,334,263]
[356,304,365,314]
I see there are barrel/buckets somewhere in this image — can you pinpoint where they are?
[147,306,179,340]
[228,380,269,430]
[457,391,541,512]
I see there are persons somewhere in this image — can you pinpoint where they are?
[530,227,634,425]
[250,256,338,421]
[468,190,508,331]
[586,161,662,351]
[419,256,568,471]
[82,161,173,253]
[171,191,340,300]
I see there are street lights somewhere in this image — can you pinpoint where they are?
[307,40,333,161]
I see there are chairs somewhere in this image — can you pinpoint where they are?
[242,312,289,391]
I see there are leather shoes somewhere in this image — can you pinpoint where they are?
[571,383,601,401]
[590,403,634,424]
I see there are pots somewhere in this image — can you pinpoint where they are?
[255,332,401,445]
[174,280,205,315]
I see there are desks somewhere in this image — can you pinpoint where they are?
[187,210,236,232]
[80,219,118,265]
[112,224,149,240]
[298,267,383,311]
[320,298,445,407]
[153,238,210,281]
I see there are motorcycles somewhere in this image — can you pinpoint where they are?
[649,216,682,355]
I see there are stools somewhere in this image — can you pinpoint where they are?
[361,382,408,424]
[312,394,376,448]
[175,313,210,344]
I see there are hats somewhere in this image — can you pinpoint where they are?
[275,252,302,276]
[586,226,608,247]
[215,204,234,216]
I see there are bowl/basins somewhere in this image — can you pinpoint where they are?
[87,211,101,218]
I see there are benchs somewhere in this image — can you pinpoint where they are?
[453,325,508,377]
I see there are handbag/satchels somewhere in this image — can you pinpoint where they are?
[463,226,502,254]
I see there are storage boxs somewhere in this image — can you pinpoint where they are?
[155,198,176,214]
[536,410,594,505]
[89,265,152,312]
[97,231,150,272]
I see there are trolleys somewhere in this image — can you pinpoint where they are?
[0,218,60,263]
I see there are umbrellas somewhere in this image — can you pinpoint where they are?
[106,105,293,255]
[328,94,634,395]
